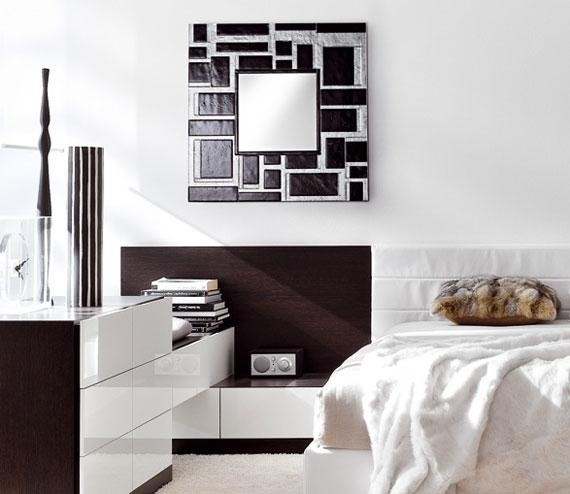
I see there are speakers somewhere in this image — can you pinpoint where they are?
[250,347,304,380]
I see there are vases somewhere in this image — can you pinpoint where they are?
[35,216,52,304]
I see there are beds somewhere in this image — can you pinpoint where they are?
[301,248,570,494]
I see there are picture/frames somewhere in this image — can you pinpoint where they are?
[233,68,325,155]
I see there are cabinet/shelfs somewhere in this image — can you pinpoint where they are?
[1,294,238,494]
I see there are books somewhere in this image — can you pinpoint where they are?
[141,277,230,332]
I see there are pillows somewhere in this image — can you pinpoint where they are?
[430,273,560,326]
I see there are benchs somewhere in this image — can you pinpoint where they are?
[172,369,334,454]
